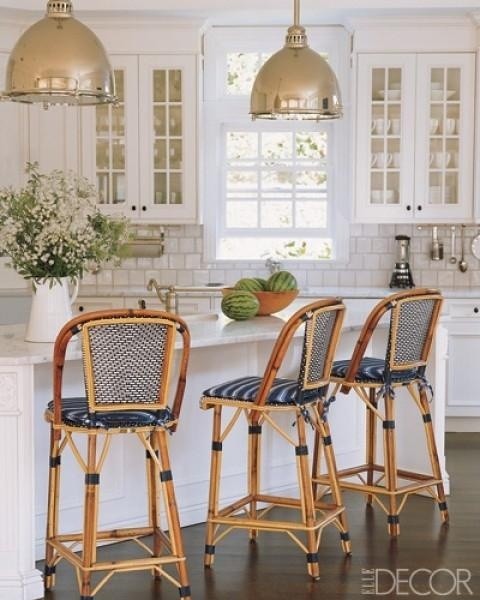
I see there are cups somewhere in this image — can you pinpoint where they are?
[99,80,182,203]
[429,185,451,204]
[431,118,460,135]
[431,151,458,169]
[371,190,395,204]
[371,118,399,135]
[373,150,398,168]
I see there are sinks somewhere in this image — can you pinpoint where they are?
[173,313,223,324]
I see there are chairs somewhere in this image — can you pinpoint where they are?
[201,297,352,582]
[41,308,193,600]
[309,285,448,541]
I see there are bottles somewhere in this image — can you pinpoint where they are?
[391,234,413,288]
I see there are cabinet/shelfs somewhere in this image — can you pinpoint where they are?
[441,294,480,417]
[78,54,198,227]
[70,298,210,318]
[353,51,475,225]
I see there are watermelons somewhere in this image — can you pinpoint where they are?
[221,271,297,321]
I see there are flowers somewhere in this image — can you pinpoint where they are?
[0,159,134,289]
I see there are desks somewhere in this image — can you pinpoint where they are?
[1,298,452,599]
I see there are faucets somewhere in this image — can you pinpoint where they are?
[146,279,177,313]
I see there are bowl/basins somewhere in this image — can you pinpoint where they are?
[432,81,443,90]
[379,89,401,99]
[432,90,457,101]
[223,289,300,318]
[389,82,401,89]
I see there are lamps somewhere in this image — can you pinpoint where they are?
[2,0,121,109]
[246,0,345,123]
